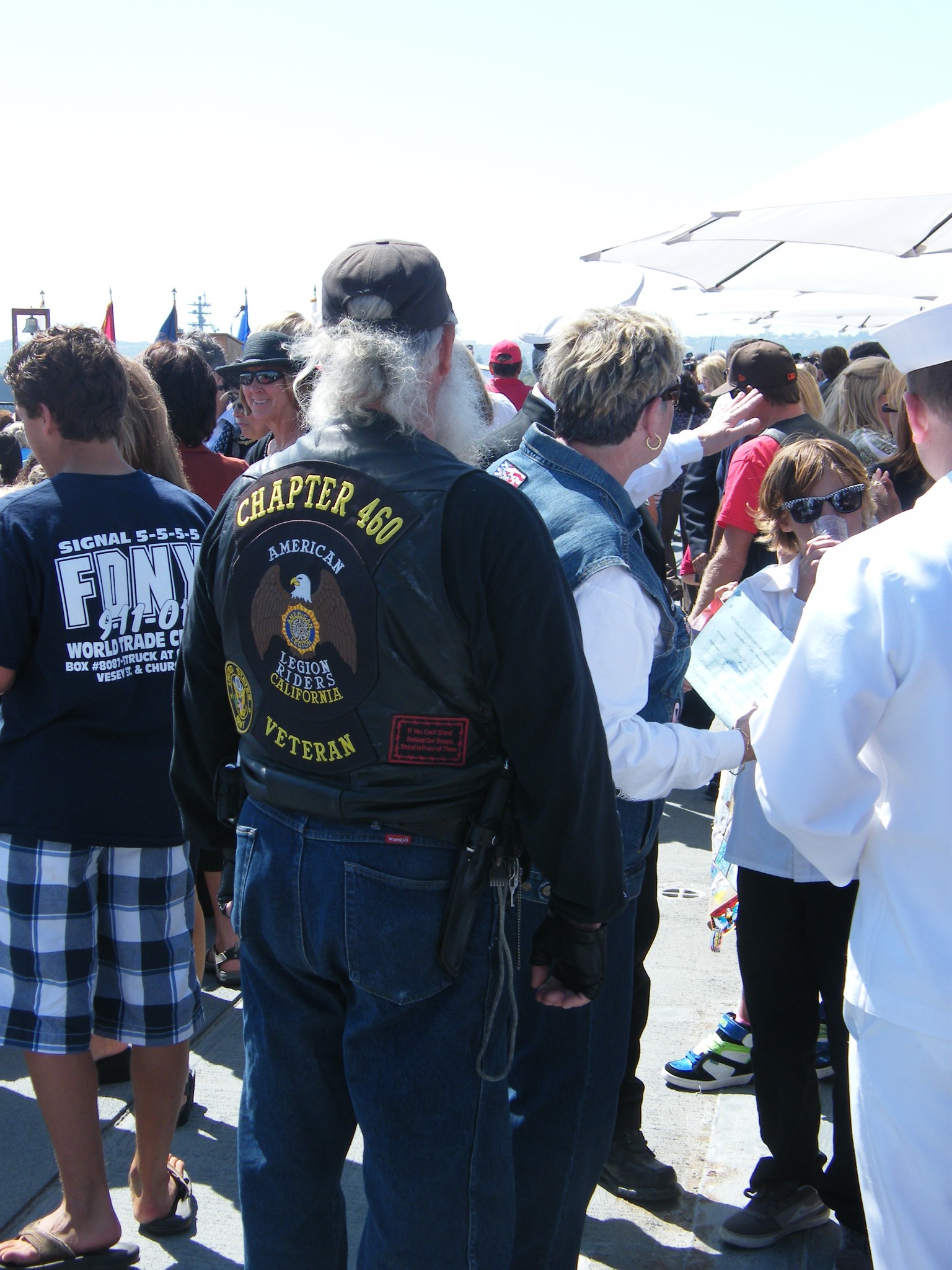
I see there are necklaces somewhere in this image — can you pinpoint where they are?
[281,432,293,446]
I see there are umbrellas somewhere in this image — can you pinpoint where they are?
[579,195,952,333]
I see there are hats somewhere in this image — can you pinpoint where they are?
[710,340,796,397]
[802,356,820,369]
[322,239,458,334]
[872,303,952,375]
[490,339,521,364]
[215,331,302,390]
[850,342,889,359]
[694,353,708,360]
[517,308,575,350]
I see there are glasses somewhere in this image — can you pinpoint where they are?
[644,375,681,406]
[230,399,244,410]
[781,483,865,524]
[238,370,284,385]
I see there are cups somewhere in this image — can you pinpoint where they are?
[808,514,849,543]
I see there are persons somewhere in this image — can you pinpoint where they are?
[1,239,951,1269]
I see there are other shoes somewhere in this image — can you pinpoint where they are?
[598,1128,677,1200]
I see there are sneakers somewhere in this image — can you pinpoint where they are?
[720,1188,829,1248]
[663,1011,755,1089]
[835,1212,872,1270]
[816,1023,833,1079]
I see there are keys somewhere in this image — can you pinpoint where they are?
[509,857,519,907]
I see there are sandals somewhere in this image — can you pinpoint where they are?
[205,943,241,983]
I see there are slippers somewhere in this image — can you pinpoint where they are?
[146,1164,191,1236]
[0,1220,140,1270]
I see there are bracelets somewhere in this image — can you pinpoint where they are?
[727,727,750,775]
